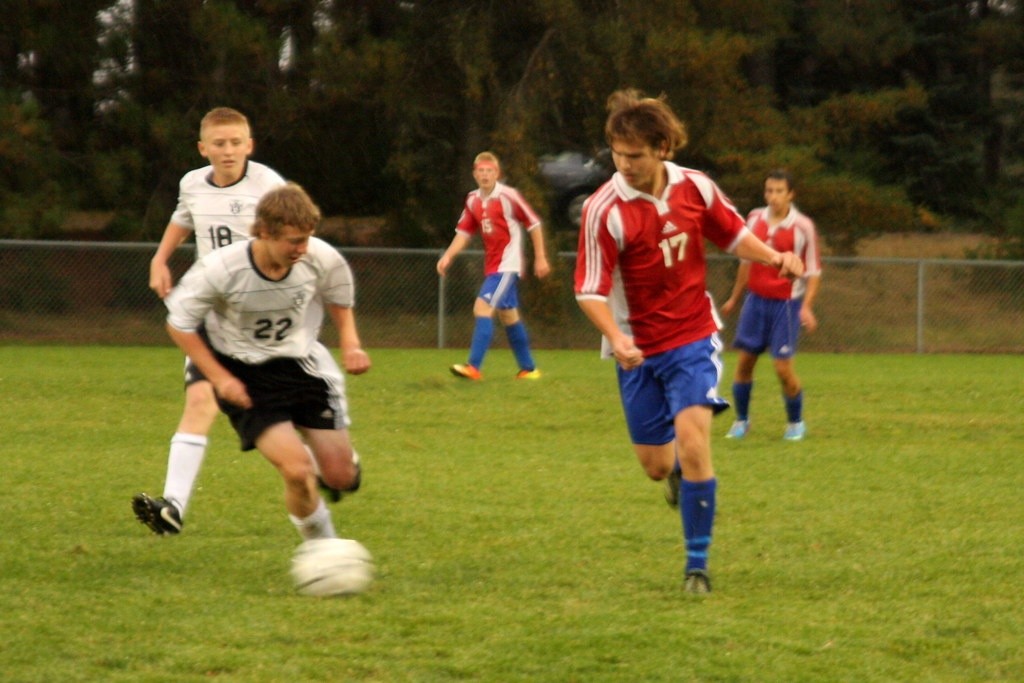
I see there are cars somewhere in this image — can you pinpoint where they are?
[539,151,607,229]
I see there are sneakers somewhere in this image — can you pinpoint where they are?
[512,369,541,378]
[783,420,804,440]
[315,472,341,503]
[131,492,183,536]
[723,420,751,439]
[343,449,361,491]
[683,572,711,594]
[664,468,680,506]
[450,363,481,380]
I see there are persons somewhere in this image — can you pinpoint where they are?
[129,107,291,535]
[434,152,551,383]
[719,166,824,443]
[164,185,370,539]
[573,88,805,600]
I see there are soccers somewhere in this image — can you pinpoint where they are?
[287,537,378,598]
